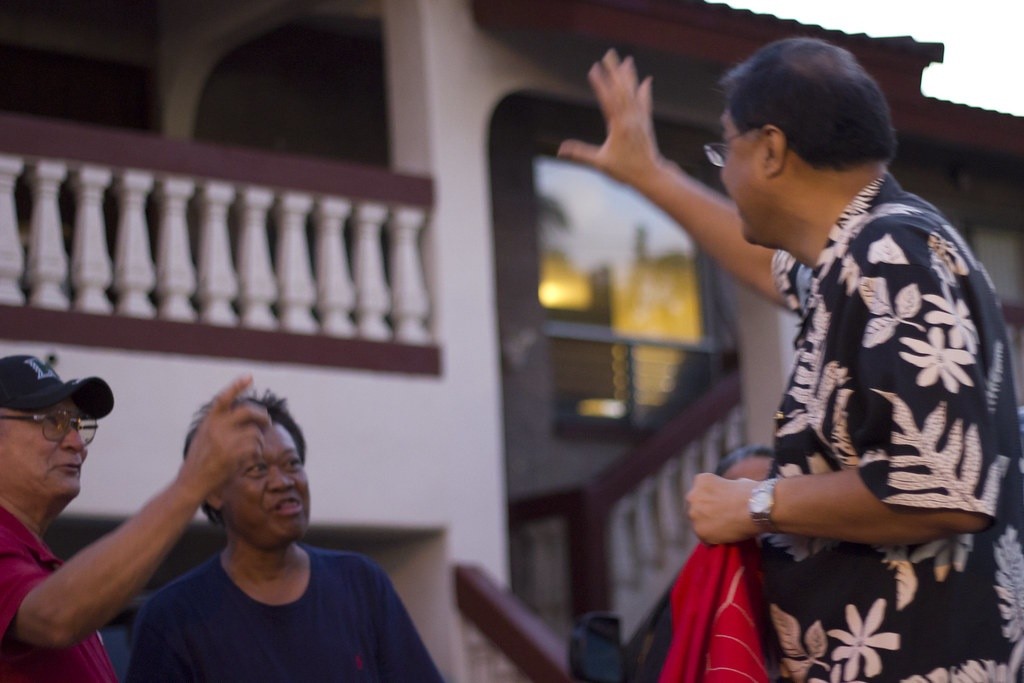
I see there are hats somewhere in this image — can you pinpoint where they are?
[0,354,115,419]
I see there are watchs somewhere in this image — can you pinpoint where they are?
[748,477,779,533]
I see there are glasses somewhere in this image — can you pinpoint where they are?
[0,409,99,447]
[704,124,765,168]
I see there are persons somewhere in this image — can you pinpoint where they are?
[1,355,272,683]
[556,39,1024,683]
[124,387,446,683]
